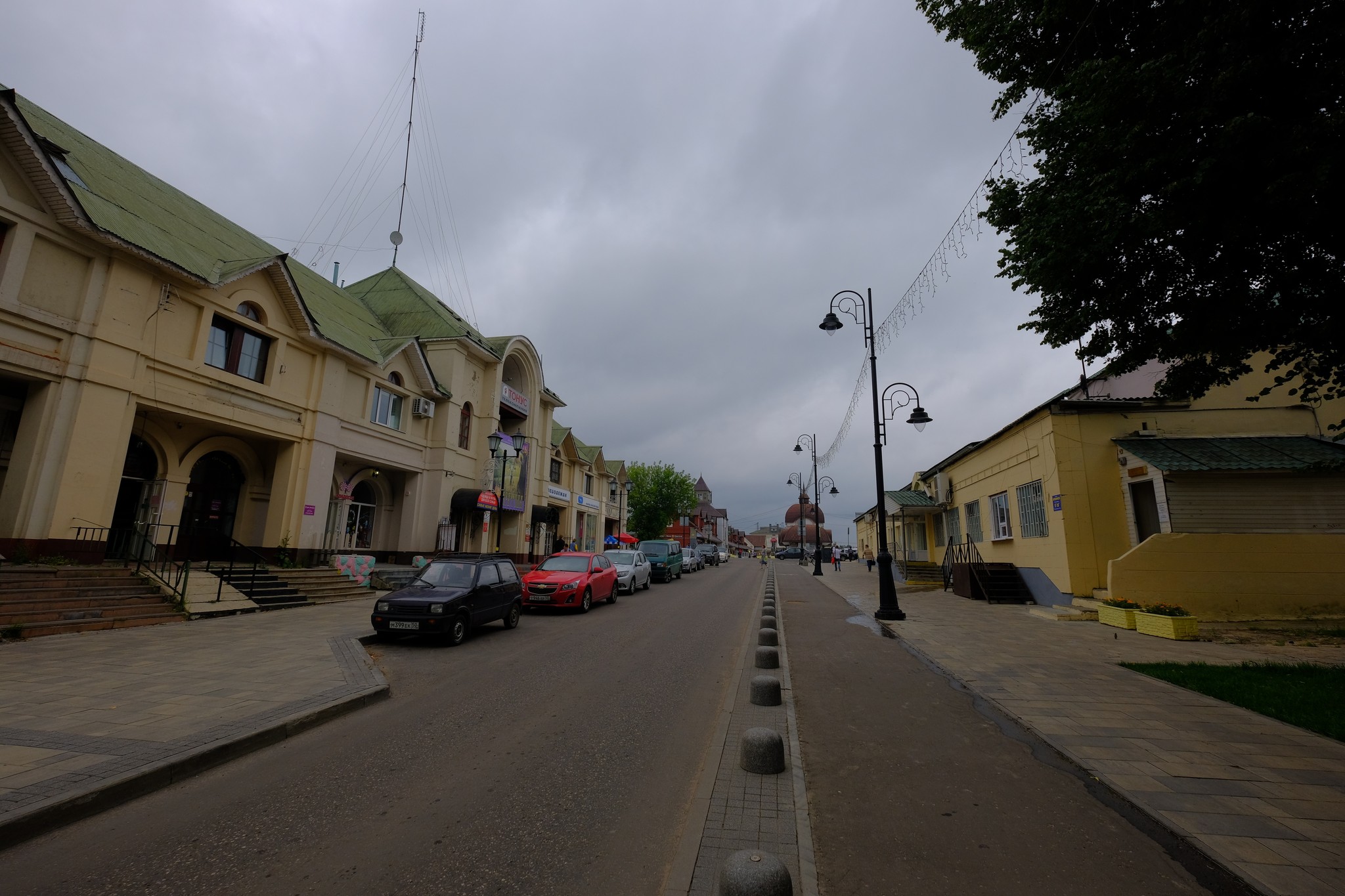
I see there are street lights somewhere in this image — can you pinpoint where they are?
[817,286,934,620]
[486,427,528,553]
[786,472,816,566]
[792,433,840,576]
[609,478,633,549]
[703,516,714,544]
[677,507,691,548]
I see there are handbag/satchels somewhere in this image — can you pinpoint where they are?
[832,557,835,565]
[871,560,876,566]
[763,557,768,564]
[834,557,836,562]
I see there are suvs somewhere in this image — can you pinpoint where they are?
[695,543,720,566]
[371,551,524,646]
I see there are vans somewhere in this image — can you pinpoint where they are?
[839,548,858,556]
[634,539,684,583]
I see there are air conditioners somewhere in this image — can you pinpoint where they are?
[864,513,874,525]
[933,472,951,506]
[411,396,435,419]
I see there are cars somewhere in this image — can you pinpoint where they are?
[601,549,652,596]
[774,547,814,560]
[520,552,618,614]
[839,552,849,561]
[717,546,730,563]
[680,548,706,573]
[851,550,858,561]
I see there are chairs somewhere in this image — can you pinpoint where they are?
[444,566,500,587]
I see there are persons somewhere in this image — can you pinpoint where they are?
[752,551,757,558]
[830,544,836,558]
[775,550,778,553]
[554,534,565,553]
[686,543,691,549]
[561,544,572,552]
[759,545,768,569]
[770,551,774,556]
[832,545,841,571]
[517,445,527,501]
[862,545,874,572]
[739,548,742,558]
[847,546,854,562]
[569,538,578,552]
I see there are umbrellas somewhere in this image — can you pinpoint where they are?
[604,535,626,545]
[612,532,639,543]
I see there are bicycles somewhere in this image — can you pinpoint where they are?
[799,552,816,566]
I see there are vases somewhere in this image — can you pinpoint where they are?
[1097,604,1147,630]
[1132,611,1199,641]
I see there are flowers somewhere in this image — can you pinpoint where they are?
[1139,601,1193,618]
[1100,596,1144,610]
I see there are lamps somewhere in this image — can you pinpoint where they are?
[372,467,381,478]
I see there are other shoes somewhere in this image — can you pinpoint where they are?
[765,567,768,568]
[759,567,762,569]
[838,568,841,571]
[868,571,871,572]
[834,570,837,571]
